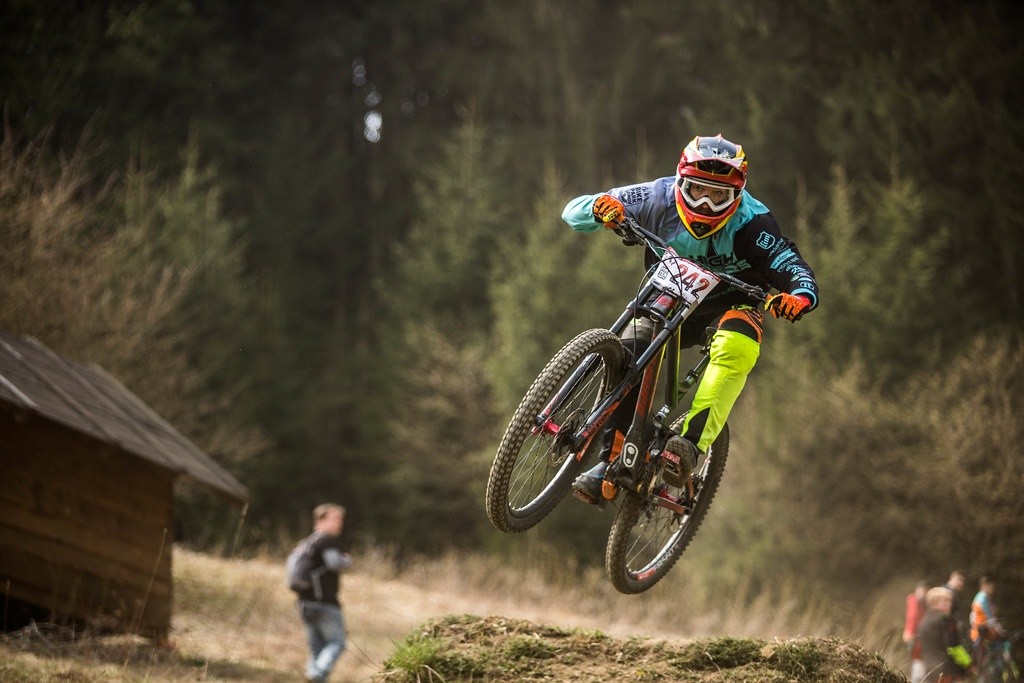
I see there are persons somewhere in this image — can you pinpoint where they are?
[561,135,819,511]
[285,502,364,683]
[944,569,970,644]
[901,579,930,683]
[969,572,1010,654]
[910,586,984,683]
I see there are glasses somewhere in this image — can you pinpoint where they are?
[682,178,737,213]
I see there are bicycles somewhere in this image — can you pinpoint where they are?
[482,198,803,594]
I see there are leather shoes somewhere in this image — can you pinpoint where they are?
[574,461,620,513]
[662,436,698,490]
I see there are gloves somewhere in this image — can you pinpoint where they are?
[592,195,624,229]
[763,292,811,324]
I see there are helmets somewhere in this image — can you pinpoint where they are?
[675,135,748,240]
